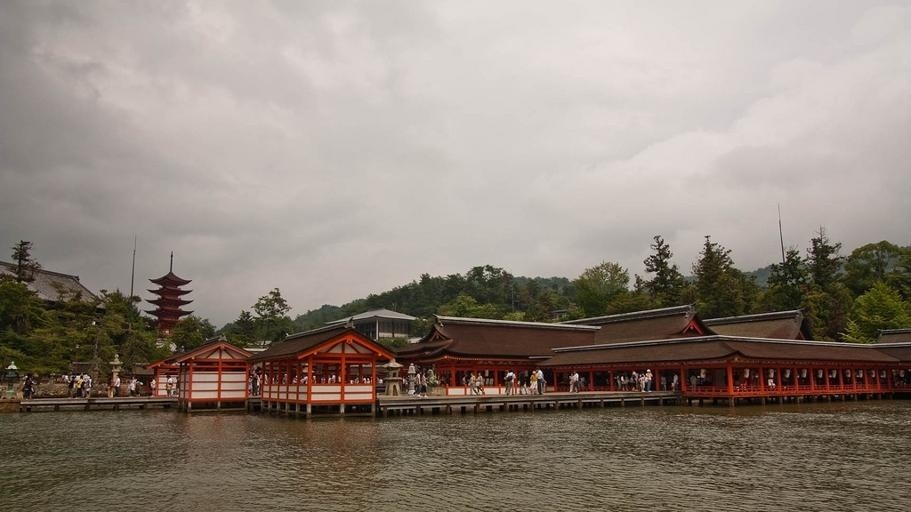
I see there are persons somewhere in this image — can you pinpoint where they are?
[768,378,777,389]
[127,375,139,396]
[149,376,157,397]
[249,366,410,397]
[468,372,479,396]
[572,370,581,393]
[476,372,486,396]
[569,372,575,392]
[15,374,37,401]
[421,371,429,398]
[413,370,423,398]
[167,382,173,397]
[503,367,549,397]
[166,374,174,395]
[60,371,94,398]
[614,369,699,394]
[112,374,122,396]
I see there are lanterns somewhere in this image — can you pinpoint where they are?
[859,369,865,378]
[801,368,808,378]
[846,369,852,379]
[832,368,838,378]
[769,367,776,378]
[784,368,792,378]
[742,368,751,379]
[871,369,876,378]
[701,368,708,380]
[900,369,905,377]
[882,369,888,379]
[817,369,824,378]
